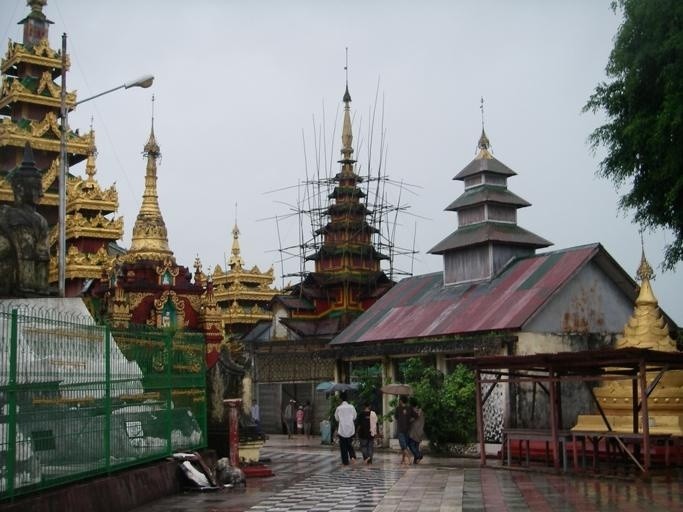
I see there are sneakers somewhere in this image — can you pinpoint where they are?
[339,454,423,470]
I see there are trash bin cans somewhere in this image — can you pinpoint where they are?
[319,420,332,445]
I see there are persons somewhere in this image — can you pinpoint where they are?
[407,401,425,467]
[285,398,313,440]
[250,399,260,439]
[355,403,379,467]
[162,311,171,325]
[394,396,417,466]
[334,394,358,468]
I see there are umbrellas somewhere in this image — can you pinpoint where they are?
[326,383,358,393]
[380,384,413,395]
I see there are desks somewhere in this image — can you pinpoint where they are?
[501,425,672,475]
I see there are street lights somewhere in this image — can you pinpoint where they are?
[57,33,157,299]
